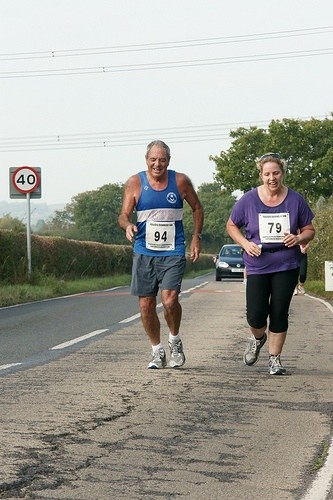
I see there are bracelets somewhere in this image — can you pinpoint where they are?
[192,234,202,240]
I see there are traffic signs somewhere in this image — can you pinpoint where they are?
[9,166,42,275]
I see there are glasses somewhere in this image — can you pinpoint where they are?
[259,153,281,161]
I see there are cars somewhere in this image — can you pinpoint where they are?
[212,244,245,281]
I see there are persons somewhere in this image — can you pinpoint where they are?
[293,229,310,295]
[226,152,315,374]
[118,140,205,370]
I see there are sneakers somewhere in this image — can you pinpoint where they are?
[168,340,185,368]
[148,348,167,369]
[268,354,287,375]
[293,286,297,296]
[243,333,267,366]
[297,284,305,294]
